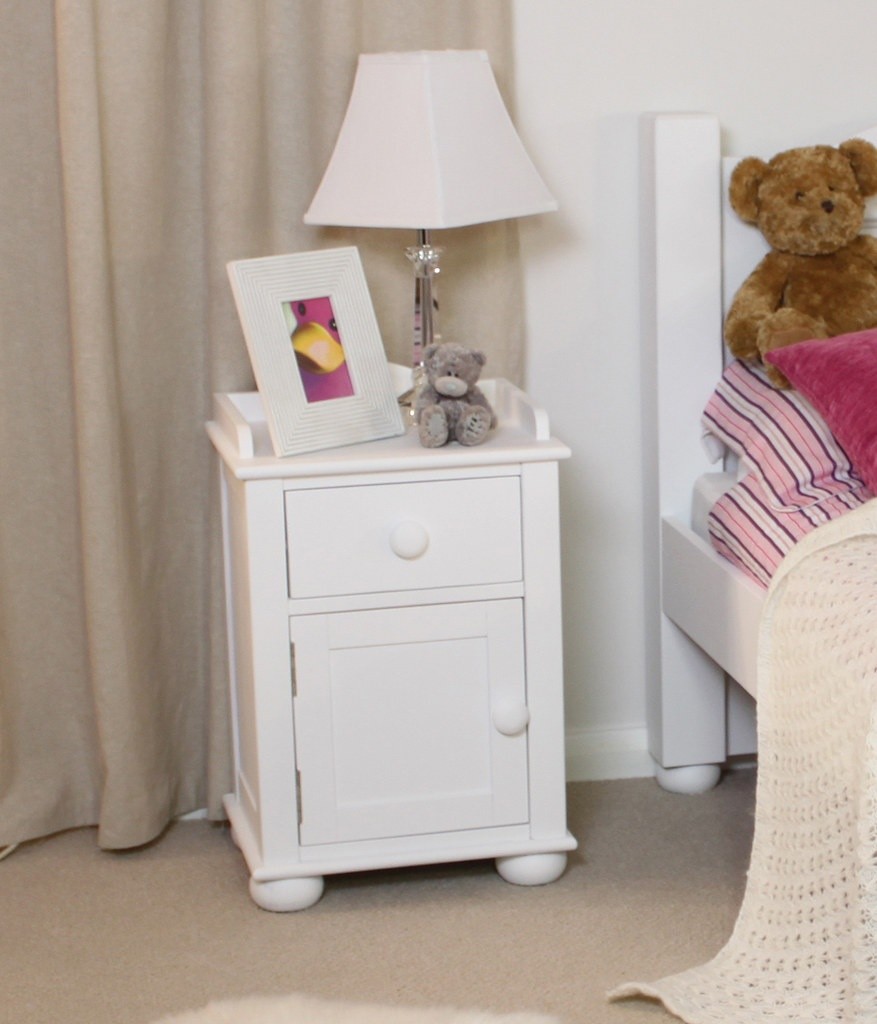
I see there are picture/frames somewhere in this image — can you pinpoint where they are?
[226,245,405,459]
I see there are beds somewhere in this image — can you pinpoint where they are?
[610,106,877,1024]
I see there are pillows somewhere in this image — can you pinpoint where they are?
[701,356,866,513]
[764,327,877,499]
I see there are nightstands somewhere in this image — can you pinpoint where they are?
[201,414,579,912]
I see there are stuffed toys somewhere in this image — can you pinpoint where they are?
[725,138,877,389]
[414,341,497,448]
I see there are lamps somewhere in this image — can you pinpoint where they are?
[305,48,562,409]
